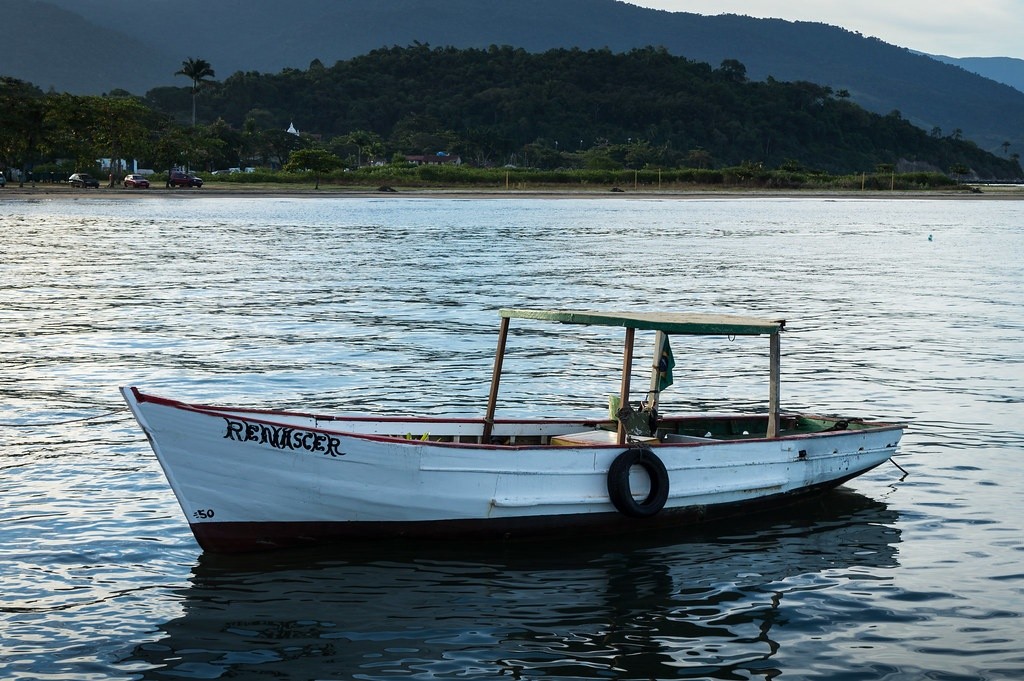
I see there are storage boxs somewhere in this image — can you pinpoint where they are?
[552,430,660,445]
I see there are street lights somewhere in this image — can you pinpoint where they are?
[581,140,583,151]
[555,140,558,149]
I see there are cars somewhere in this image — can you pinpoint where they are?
[68,173,99,189]
[0,172,6,187]
[124,174,150,188]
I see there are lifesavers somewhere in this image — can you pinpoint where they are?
[607,449,670,519]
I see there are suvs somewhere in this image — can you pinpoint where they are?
[171,172,204,187]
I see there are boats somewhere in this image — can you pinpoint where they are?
[119,309,907,563]
